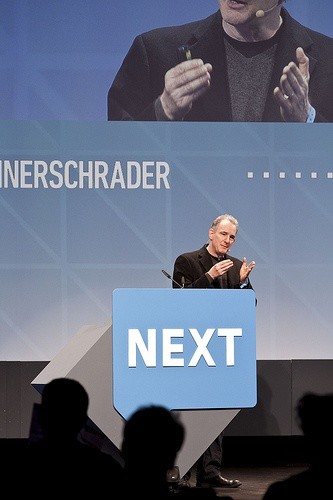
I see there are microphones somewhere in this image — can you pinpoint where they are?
[162,270,184,288]
[228,249,230,252]
[256,4,278,17]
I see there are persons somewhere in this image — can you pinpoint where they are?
[0,378,218,500]
[260,391,333,500]
[107,1,333,123]
[172,214,258,488]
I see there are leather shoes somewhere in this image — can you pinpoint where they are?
[178,479,190,487]
[197,474,242,488]
